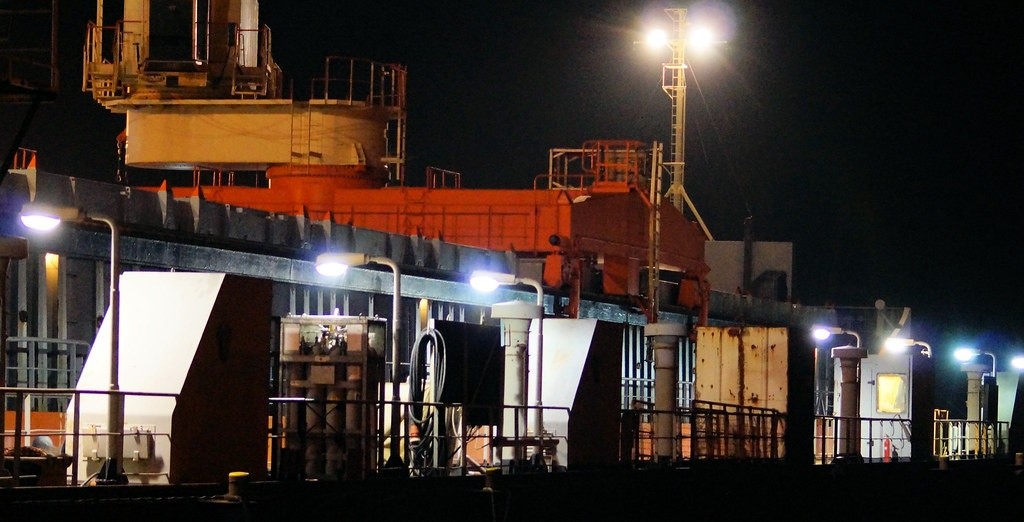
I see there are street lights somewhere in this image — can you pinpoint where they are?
[15,200,129,487]
[316,250,408,476]
[952,348,999,462]
[468,268,548,470]
[886,338,941,467]
[811,321,862,462]
